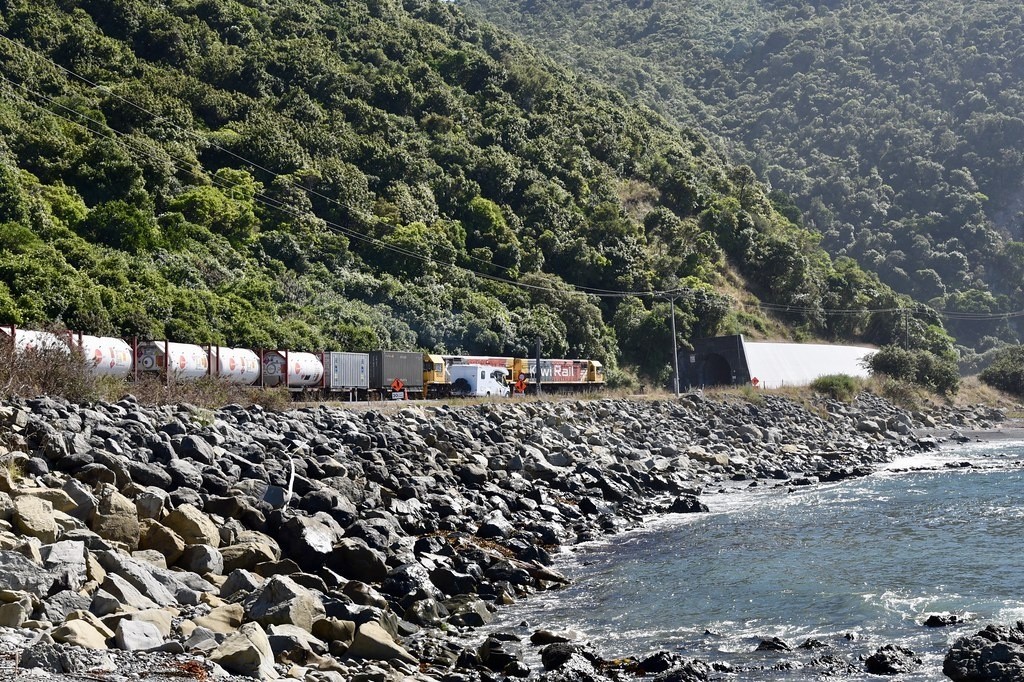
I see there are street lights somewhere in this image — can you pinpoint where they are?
[654,287,695,397]
[891,307,911,351]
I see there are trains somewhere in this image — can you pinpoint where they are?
[0,323,605,405]
